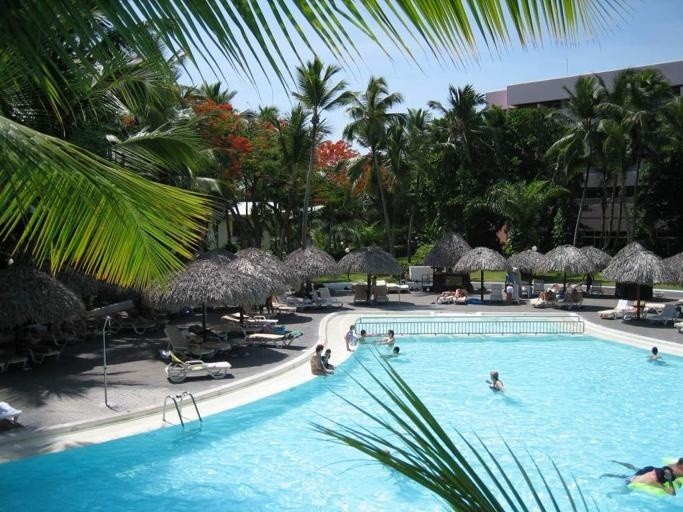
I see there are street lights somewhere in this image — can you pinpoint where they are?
[101,310,131,406]
[341,238,351,280]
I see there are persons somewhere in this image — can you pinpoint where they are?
[648,347,665,366]
[486,371,503,392]
[605,465,677,497]
[601,451,683,487]
[311,320,400,377]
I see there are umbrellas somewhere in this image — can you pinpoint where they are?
[141,241,403,345]
[424,230,683,324]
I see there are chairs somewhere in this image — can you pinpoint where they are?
[0,401,22,423]
[161,278,345,382]
[351,283,392,306]
[529,280,587,311]
[597,295,682,327]
[431,287,470,307]
